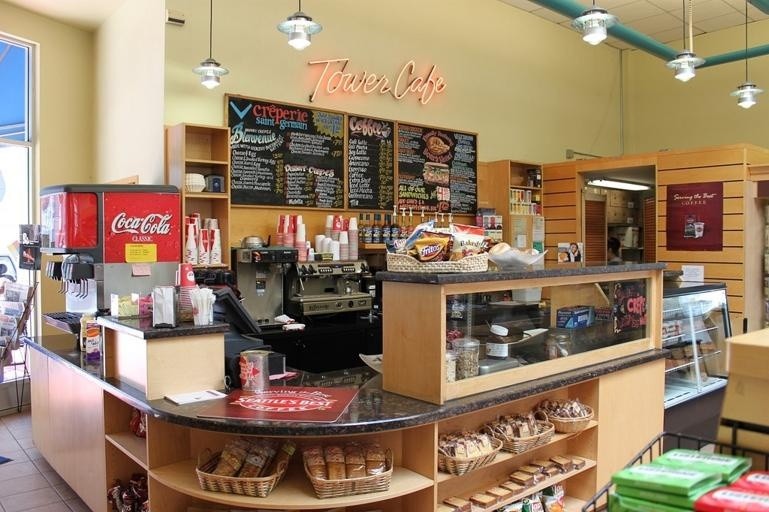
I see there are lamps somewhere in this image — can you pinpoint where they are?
[586,178,652,191]
[729,1,763,115]
[574,1,619,49]
[278,2,323,52]
[662,2,704,85]
[190,2,229,92]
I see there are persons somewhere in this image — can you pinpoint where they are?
[489,217,496,229]
[607,237,623,262]
[559,243,581,261]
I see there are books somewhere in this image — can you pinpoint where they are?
[0,281,34,382]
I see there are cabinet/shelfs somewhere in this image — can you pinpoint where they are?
[407,379,597,512]
[662,284,733,451]
[482,158,546,259]
[101,391,147,512]
[147,417,435,512]
[164,122,233,270]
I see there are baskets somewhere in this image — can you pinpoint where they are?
[194,443,288,498]
[437,430,503,477]
[386,248,488,274]
[304,446,393,499]
[577,432,768,512]
[485,412,555,453]
[538,397,594,432]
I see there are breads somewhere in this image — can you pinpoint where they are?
[306,446,387,480]
[214,439,272,477]
[443,454,586,511]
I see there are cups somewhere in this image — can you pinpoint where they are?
[240,349,269,389]
[695,222,704,238]
[183,212,223,264]
[279,213,362,265]
[178,263,198,322]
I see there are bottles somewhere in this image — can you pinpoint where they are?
[487,325,509,359]
[359,211,456,244]
[79,313,93,349]
[86,321,102,359]
[454,338,480,379]
[546,330,571,361]
[445,351,456,383]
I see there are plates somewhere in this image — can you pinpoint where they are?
[486,250,547,270]
[185,173,206,192]
